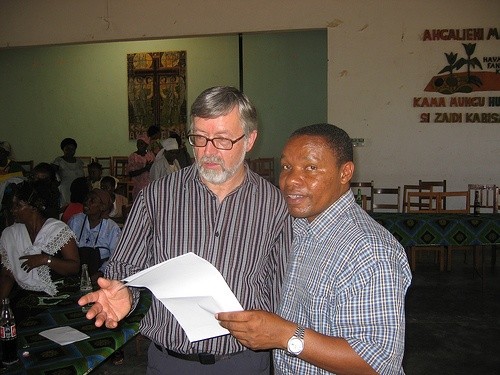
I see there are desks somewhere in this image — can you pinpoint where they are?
[368,212,500,247]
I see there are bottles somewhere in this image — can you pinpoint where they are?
[0,298,18,364]
[474,191,480,215]
[356,189,362,207]
[80,264,94,312]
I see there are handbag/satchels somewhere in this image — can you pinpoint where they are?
[78,246,111,277]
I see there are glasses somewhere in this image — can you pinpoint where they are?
[185,130,245,150]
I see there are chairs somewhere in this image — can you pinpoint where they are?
[347,179,500,278]
[95,155,127,199]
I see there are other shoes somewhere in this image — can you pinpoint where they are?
[112,346,128,366]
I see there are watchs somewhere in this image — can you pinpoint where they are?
[285,324,306,357]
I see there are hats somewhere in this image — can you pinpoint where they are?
[163,138,179,151]
[0,141,11,155]
[137,132,150,146]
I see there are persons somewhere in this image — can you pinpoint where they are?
[78,87,297,375]
[0,138,127,365]
[127,126,190,198]
[215,123,412,375]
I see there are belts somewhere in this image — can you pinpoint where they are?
[154,342,243,365]
